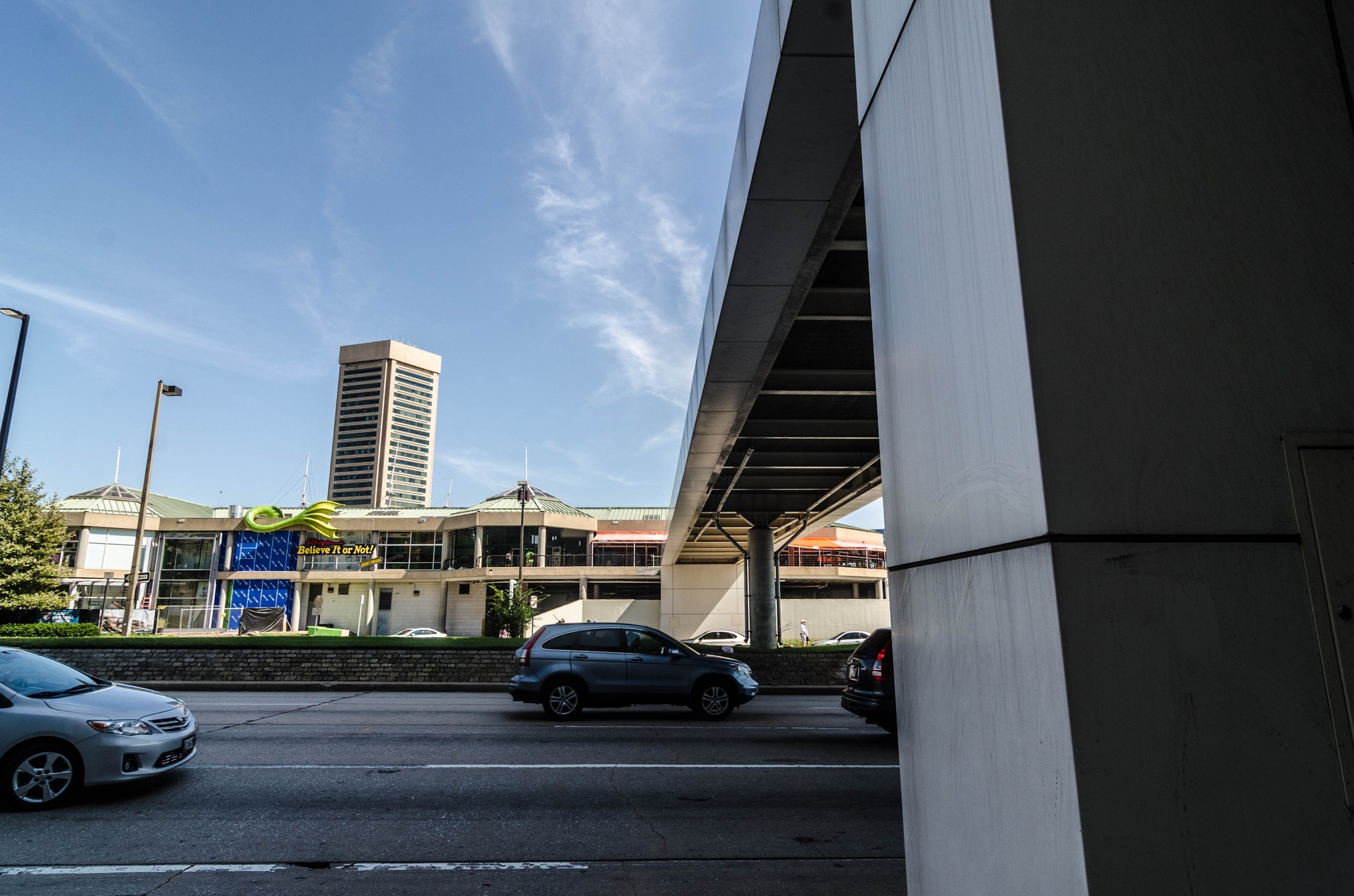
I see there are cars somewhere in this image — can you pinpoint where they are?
[386,627,448,638]
[0,645,200,813]
[810,631,871,647]
[679,629,751,647]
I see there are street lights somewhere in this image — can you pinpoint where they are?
[120,378,184,637]
[514,480,529,638]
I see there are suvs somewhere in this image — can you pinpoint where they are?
[840,627,898,737]
[507,620,761,722]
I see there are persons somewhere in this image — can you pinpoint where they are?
[486,556,492,567]
[499,624,508,638]
[525,549,537,567]
[800,620,809,647]
[505,551,513,567]
[627,631,658,656]
[606,554,611,564]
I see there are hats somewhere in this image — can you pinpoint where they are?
[800,620,807,623]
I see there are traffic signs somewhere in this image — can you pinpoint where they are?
[124,572,150,583]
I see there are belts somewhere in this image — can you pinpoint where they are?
[800,633,804,634]
